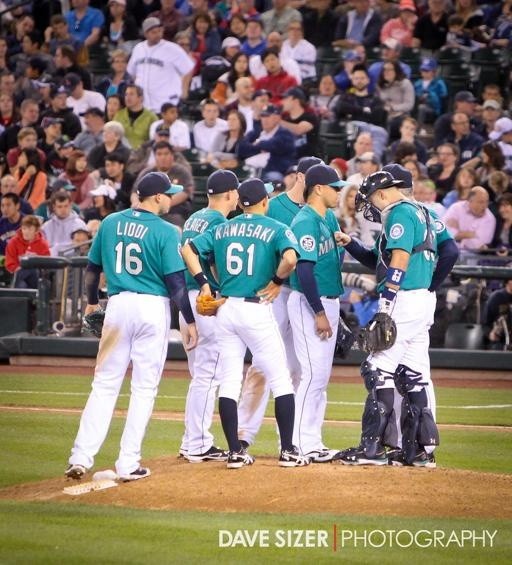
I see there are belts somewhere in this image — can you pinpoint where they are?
[320,295,339,300]
[221,295,259,303]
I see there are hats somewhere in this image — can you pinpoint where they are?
[262,170,284,182]
[238,178,270,206]
[89,184,117,199]
[52,179,77,193]
[156,124,169,135]
[137,172,183,196]
[341,1,438,69]
[455,91,512,142]
[207,169,238,194]
[40,73,105,150]
[141,17,163,32]
[221,37,241,49]
[297,151,413,197]
[252,87,305,116]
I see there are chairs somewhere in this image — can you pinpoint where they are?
[124,36,512,350]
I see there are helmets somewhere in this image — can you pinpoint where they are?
[354,172,405,223]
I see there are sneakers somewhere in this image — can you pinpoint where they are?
[64,465,86,480]
[185,445,229,462]
[278,444,311,466]
[304,447,340,462]
[331,444,437,469]
[118,467,151,482]
[227,445,256,468]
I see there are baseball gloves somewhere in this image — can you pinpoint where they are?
[196,294,226,316]
[360,312,397,352]
[83,310,106,338]
[334,310,357,358]
